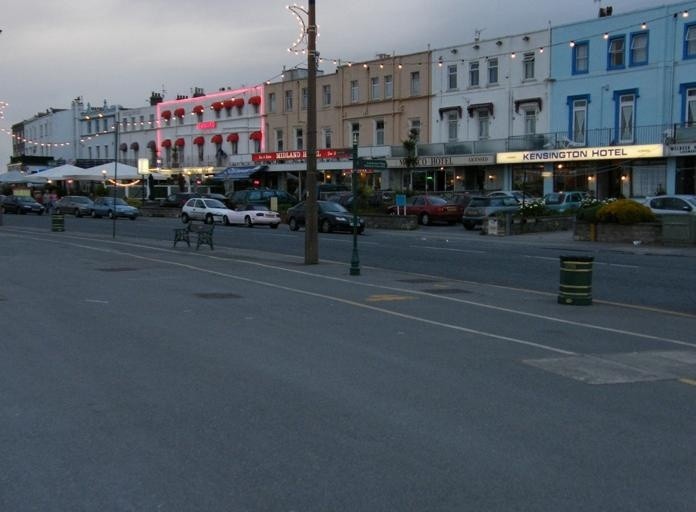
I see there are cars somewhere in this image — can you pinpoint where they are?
[446,189,588,230]
[87,197,140,221]
[52,195,95,219]
[1,195,45,217]
[159,182,391,234]
[388,195,463,228]
[642,192,696,217]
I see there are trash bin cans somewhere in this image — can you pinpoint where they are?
[558,255,594,306]
[51,213,64,232]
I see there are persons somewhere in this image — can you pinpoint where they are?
[35,189,59,215]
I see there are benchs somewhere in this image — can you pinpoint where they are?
[173,221,216,253]
